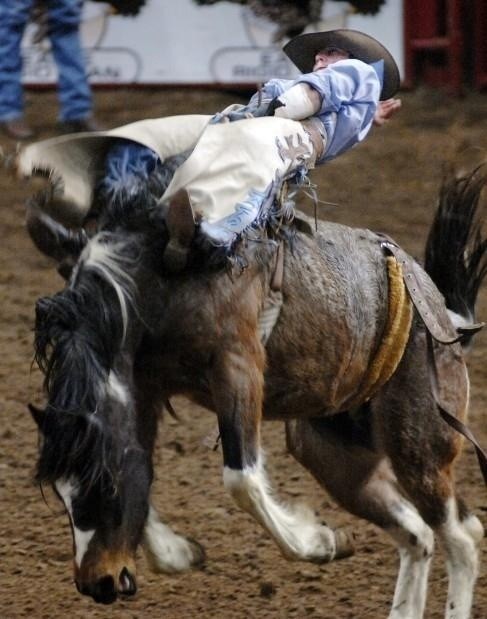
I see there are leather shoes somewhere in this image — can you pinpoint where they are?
[24,202,89,281]
[0,115,113,140]
[157,185,203,278]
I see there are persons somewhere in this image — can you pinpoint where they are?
[22,26,406,278]
[0,0,105,143]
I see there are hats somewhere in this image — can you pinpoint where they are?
[280,26,401,102]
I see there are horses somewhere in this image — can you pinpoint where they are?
[26,160,486,619]
